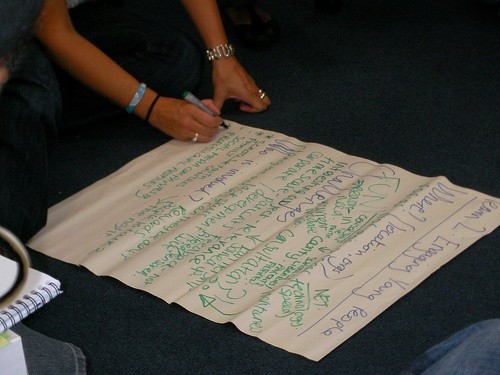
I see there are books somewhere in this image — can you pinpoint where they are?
[0,255,67,335]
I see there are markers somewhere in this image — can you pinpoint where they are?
[184,91,229,131]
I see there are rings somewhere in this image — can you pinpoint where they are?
[256,88,263,95]
[192,132,199,143]
[259,92,267,100]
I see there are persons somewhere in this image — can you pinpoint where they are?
[0,0,271,143]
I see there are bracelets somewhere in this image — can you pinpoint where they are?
[144,93,161,124]
[206,44,235,61]
[125,81,147,115]
